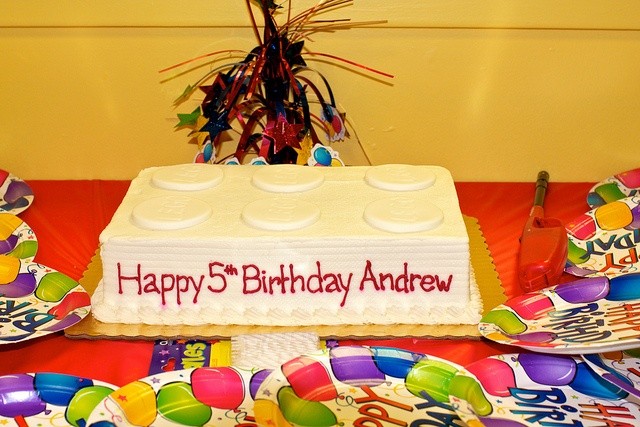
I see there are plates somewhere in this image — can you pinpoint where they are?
[479,268,639,354]
[0,255,90,343]
[557,197,640,277]
[579,352,640,399]
[0,212,39,262]
[85,368,276,427]
[586,167,640,209]
[0,168,34,216]
[255,346,466,427]
[0,373,122,427]
[449,354,640,427]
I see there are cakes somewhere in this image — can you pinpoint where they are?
[91,164,485,326]
